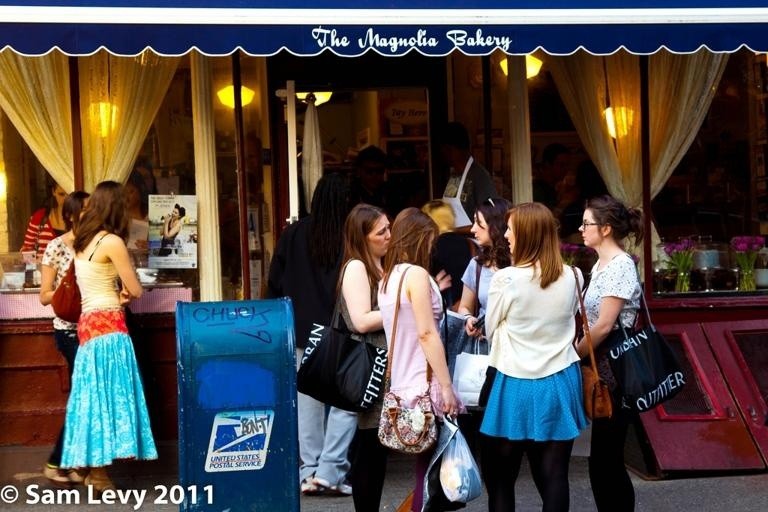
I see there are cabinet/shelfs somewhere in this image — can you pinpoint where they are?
[350,86,431,181]
[747,50,767,237]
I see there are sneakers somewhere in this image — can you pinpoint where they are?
[302,476,352,492]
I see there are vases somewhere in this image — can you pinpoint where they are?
[739,268,756,290]
[674,270,690,292]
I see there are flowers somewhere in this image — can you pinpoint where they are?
[561,243,579,267]
[662,238,696,292]
[730,236,765,290]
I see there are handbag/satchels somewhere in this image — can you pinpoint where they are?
[421,414,466,512]
[453,336,489,407]
[296,323,388,413]
[51,258,81,323]
[581,366,613,419]
[604,326,687,412]
[378,392,438,455]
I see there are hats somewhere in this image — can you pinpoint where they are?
[422,199,454,234]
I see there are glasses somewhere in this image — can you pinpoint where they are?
[583,222,600,230]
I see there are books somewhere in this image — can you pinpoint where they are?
[124,218,149,250]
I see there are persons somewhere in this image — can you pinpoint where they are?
[262,119,645,510]
[39,191,92,492]
[133,156,160,194]
[158,204,187,258]
[19,178,66,284]
[59,180,159,500]
[115,167,152,277]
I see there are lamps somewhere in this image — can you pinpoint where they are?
[499,55,543,79]
[292,90,333,107]
[218,81,256,111]
[599,97,634,139]
[86,99,121,140]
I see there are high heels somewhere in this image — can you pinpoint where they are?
[85,474,126,500]
[42,465,85,490]
